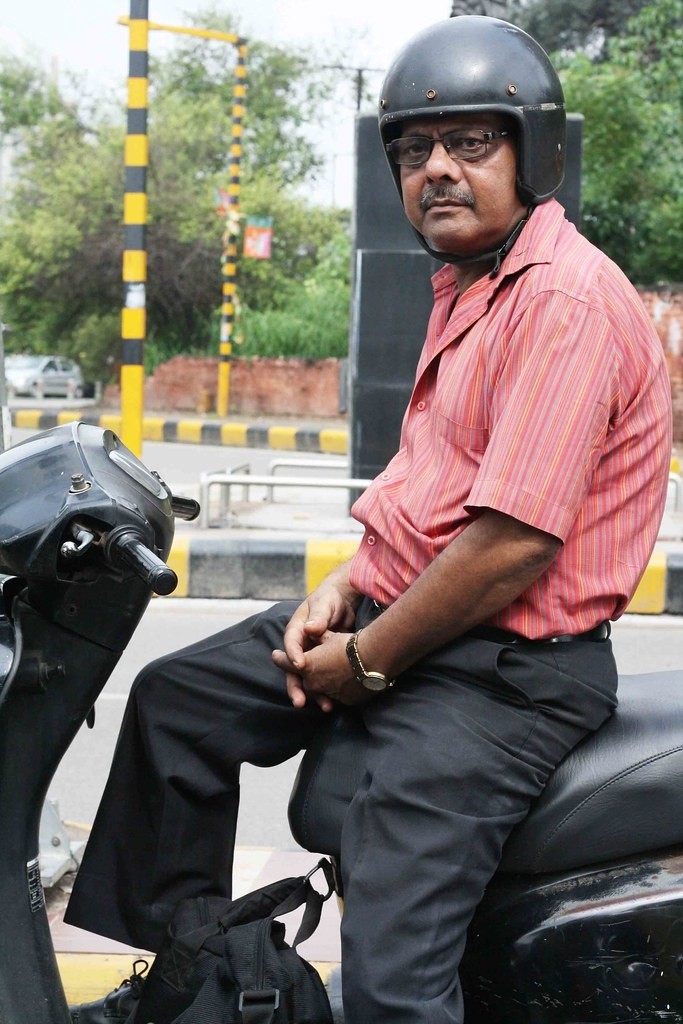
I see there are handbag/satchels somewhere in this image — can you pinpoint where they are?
[130,853,337,1023]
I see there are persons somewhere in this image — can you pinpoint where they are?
[65,15,673,1023]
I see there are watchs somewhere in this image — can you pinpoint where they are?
[346,629,397,693]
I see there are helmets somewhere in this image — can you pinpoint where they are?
[377,15,564,208]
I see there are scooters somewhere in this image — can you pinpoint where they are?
[0,421,683,1024]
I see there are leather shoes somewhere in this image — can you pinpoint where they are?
[69,959,148,1024]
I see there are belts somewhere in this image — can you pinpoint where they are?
[478,618,608,647]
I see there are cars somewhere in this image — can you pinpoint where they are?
[4,353,87,401]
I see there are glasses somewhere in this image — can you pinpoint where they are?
[383,128,515,168]
